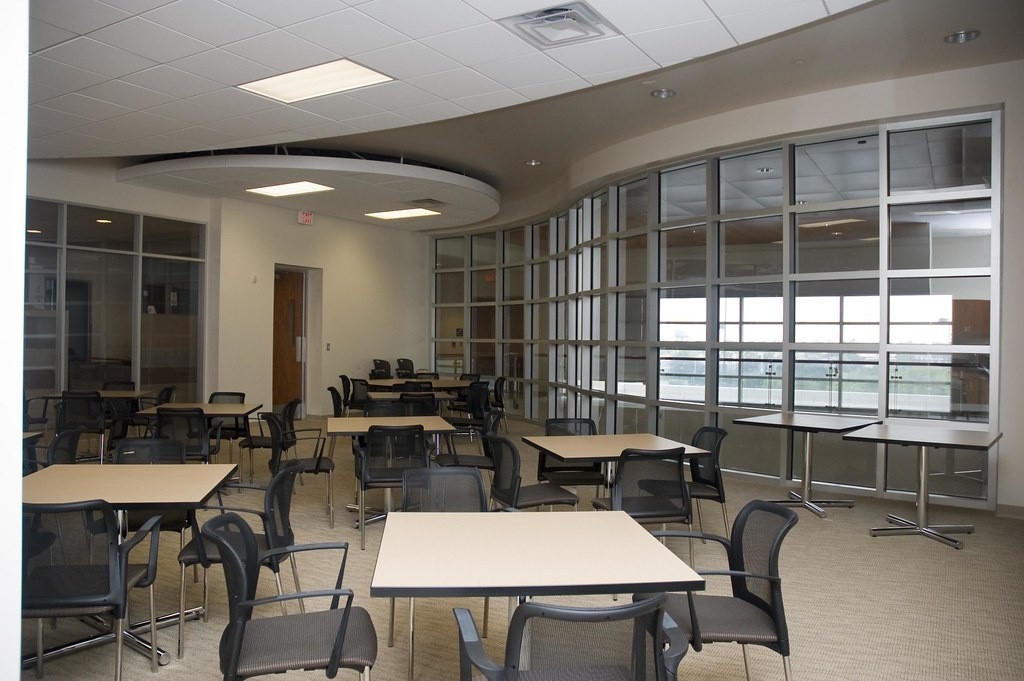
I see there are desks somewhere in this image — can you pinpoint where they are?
[22,463,238,671]
[841,424,1003,550]
[366,377,474,414]
[368,391,455,459]
[43,390,161,463]
[328,415,457,528]
[731,413,884,519]
[136,402,264,496]
[371,511,707,681]
[521,433,710,511]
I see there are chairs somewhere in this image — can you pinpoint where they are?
[22,358,798,681]
